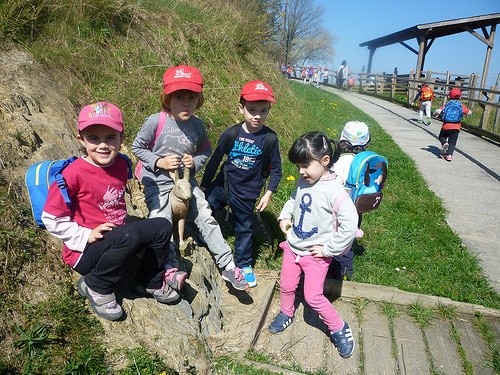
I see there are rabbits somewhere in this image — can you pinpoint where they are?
[166,158,193,247]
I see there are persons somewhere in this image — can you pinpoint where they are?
[40,102,181,320]
[269,131,359,358]
[131,65,250,290]
[329,121,371,279]
[411,82,434,126]
[433,88,472,162]
[282,59,355,93]
[199,79,283,288]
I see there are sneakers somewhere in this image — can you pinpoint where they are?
[75,275,124,321]
[267,311,293,334]
[331,320,356,358]
[163,266,189,291]
[441,143,452,161]
[240,265,257,288]
[135,282,181,305]
[222,268,249,291]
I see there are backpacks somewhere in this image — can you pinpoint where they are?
[419,86,433,100]
[24,151,134,230]
[134,110,210,180]
[441,100,464,123]
[340,150,388,213]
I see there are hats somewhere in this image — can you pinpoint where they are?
[240,80,277,105]
[339,120,370,147]
[448,88,462,98]
[77,102,124,133]
[162,65,205,94]
